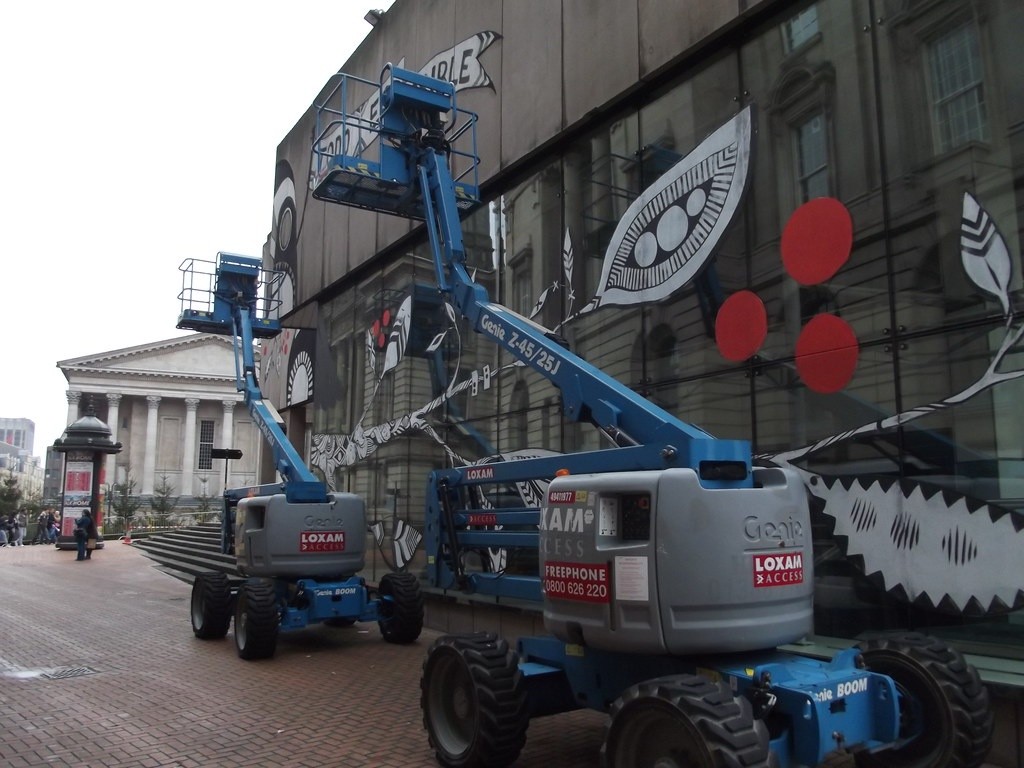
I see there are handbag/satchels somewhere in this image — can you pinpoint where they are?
[73,528,87,537]
[87,539,96,549]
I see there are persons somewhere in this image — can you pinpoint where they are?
[84,512,98,559]
[74,509,90,561]
[0,506,61,549]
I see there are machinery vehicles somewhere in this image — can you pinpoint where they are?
[303,61,998,768]
[174,252,426,660]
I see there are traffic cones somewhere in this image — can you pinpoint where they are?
[123,527,133,544]
